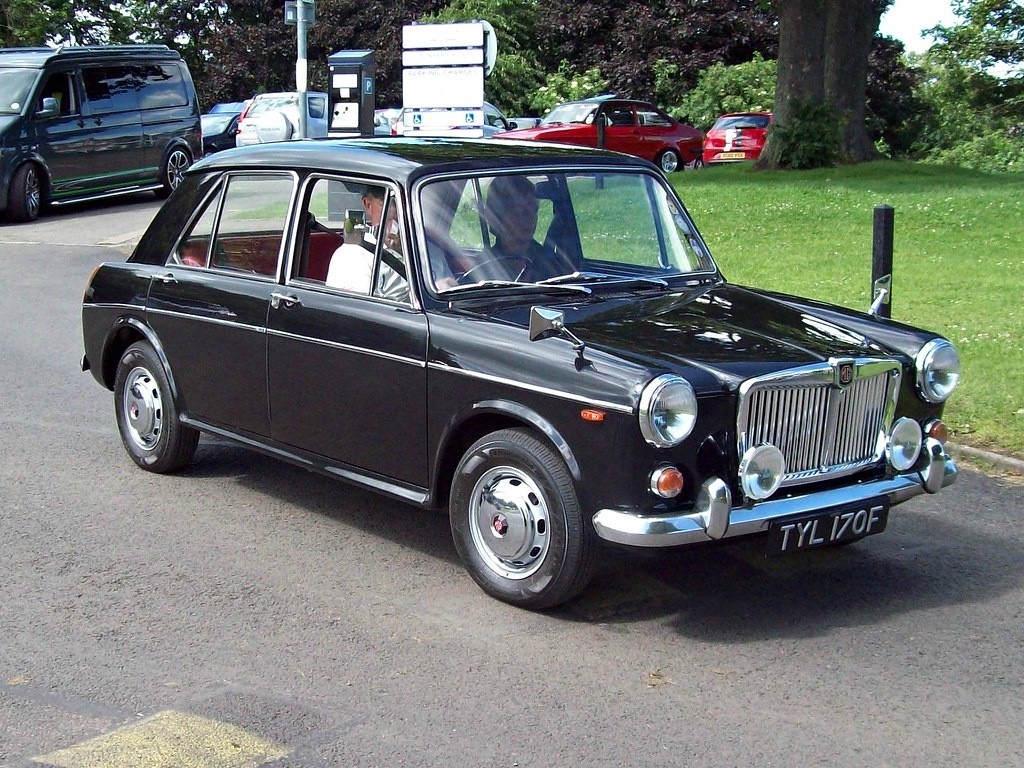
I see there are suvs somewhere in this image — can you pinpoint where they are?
[236,91,328,143]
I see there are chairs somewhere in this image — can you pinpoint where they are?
[614,107,635,124]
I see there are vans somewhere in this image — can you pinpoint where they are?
[0,44,203,225]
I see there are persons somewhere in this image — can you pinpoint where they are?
[460,175,566,285]
[326,185,459,304]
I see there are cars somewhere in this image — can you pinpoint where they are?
[79,131,963,612]
[702,112,774,168]
[391,101,519,145]
[492,94,703,174]
[200,102,247,155]
